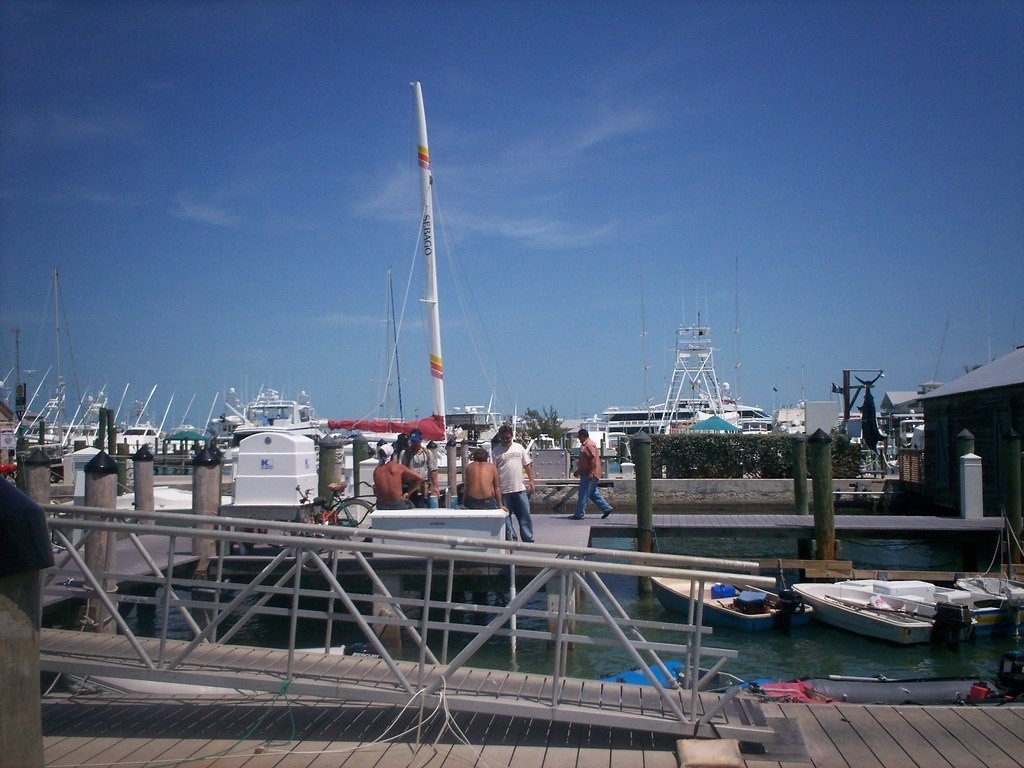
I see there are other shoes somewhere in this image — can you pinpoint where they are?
[602,508,612,519]
[570,515,585,519]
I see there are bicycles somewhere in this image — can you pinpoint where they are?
[288,482,432,571]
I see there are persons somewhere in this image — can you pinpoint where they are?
[464,425,536,543]
[373,430,440,510]
[568,429,612,520]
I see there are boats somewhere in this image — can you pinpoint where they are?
[835,578,1008,637]
[650,576,814,632]
[791,583,978,645]
[602,406,926,461]
[953,503,1024,625]
[728,612,1024,707]
[595,660,774,697]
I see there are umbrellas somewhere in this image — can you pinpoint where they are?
[690,414,741,434]
[161,432,209,449]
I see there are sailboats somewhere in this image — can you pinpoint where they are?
[0,265,491,464]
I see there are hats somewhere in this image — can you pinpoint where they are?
[409,432,422,440]
[380,443,394,458]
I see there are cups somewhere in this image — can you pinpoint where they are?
[429,496,437,508]
[451,496,458,509]
